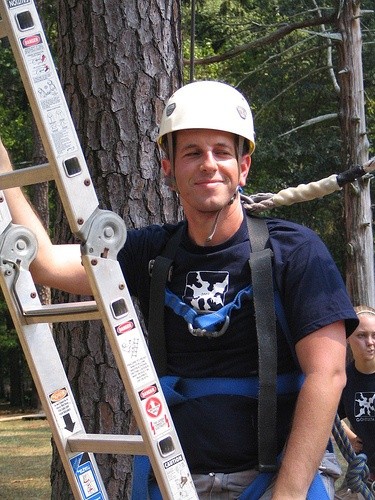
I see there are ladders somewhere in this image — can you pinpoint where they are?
[0,0,199,500]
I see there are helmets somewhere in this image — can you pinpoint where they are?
[156,79,255,161]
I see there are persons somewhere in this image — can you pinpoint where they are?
[330,305,375,499]
[2,82,360,500]
